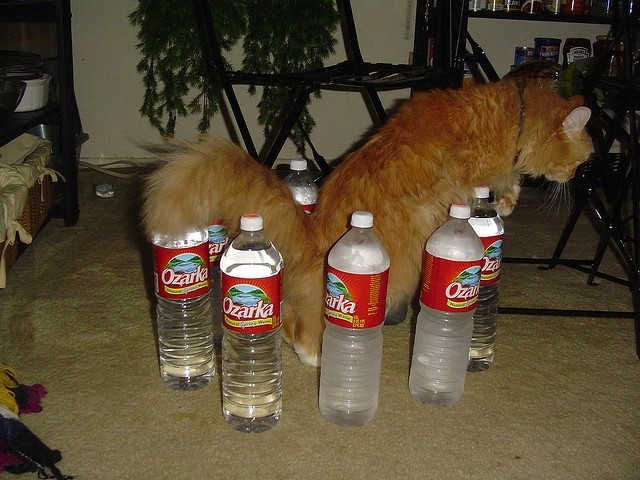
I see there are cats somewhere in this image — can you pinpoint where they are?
[140,76,596,368]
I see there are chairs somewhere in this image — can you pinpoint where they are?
[497,0,639,356]
[193,1,440,172]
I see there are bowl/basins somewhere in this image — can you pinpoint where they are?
[0,79,27,121]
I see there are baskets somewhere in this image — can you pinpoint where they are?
[16,174,52,235]
[0,239,18,269]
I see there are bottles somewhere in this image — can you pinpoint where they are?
[209,220,229,347]
[319,211,390,428]
[408,202,485,409]
[284,159,318,214]
[220,213,284,434]
[467,184,506,372]
[152,225,217,390]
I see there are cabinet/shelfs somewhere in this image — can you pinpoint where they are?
[1,1,81,273]
[412,0,639,185]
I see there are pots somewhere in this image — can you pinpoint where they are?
[14,74,52,112]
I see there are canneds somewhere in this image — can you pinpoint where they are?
[534,38,561,63]
[593,36,615,56]
